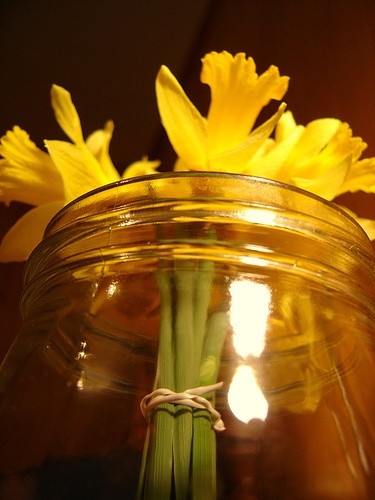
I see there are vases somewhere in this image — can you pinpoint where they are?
[1,171,374,499]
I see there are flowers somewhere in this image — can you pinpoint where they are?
[2,52,375,499]
